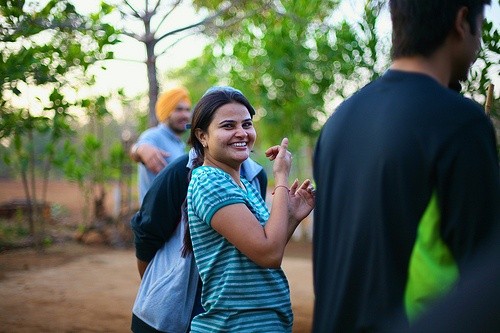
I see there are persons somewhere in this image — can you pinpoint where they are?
[130,87,192,209]
[180,91,315,333]
[309,0,500,333]
[130,85,268,333]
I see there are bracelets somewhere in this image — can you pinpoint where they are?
[270,185,291,195]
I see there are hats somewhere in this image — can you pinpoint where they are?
[155,88,191,121]
